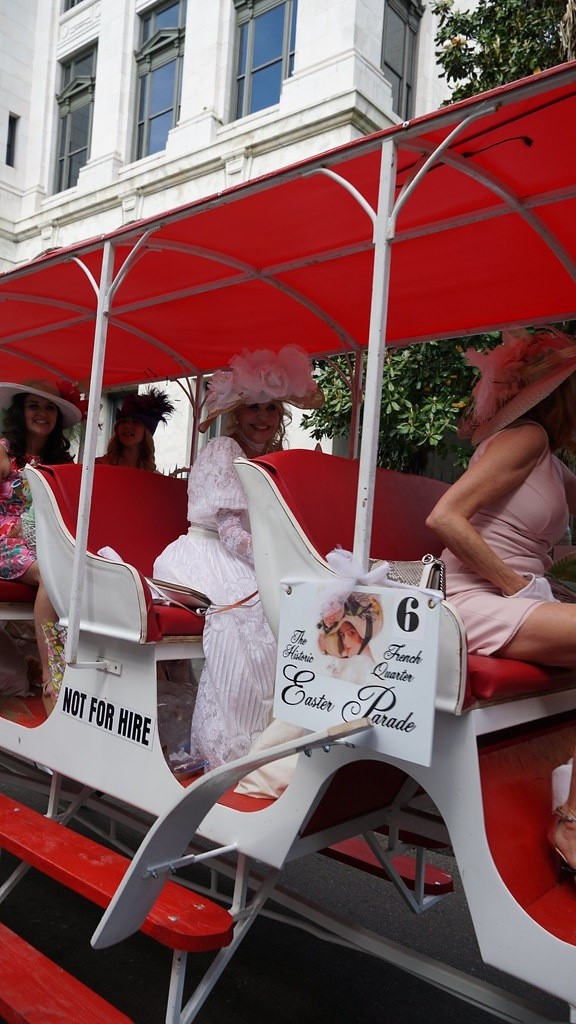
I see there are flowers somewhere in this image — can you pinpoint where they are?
[207,347,312,411]
[57,381,88,424]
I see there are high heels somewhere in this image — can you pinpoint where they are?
[42,680,57,718]
[546,807,575,883]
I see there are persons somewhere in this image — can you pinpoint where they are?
[424,321,576,885]
[317,592,384,686]
[0,379,83,717]
[92,385,176,474]
[151,342,325,801]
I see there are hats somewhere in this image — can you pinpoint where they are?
[0,380,88,429]
[457,325,576,444]
[317,592,384,658]
[114,387,175,436]
[198,344,326,433]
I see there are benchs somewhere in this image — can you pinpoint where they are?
[24,460,204,641]
[236,446,576,729]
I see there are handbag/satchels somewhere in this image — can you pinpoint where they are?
[369,554,447,602]
